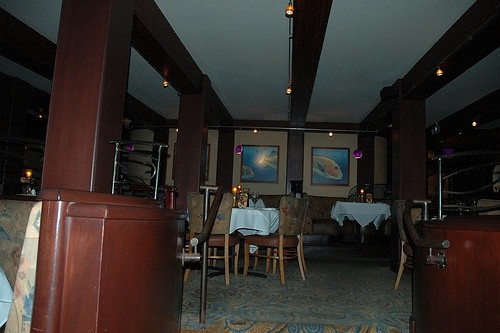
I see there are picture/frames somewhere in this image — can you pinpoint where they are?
[311,147,350,186]
[240,145,280,183]
[172,143,211,181]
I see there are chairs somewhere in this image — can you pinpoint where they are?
[243,196,310,285]
[183,191,240,285]
[393,200,428,291]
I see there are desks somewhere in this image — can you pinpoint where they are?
[329,201,391,251]
[208,207,280,274]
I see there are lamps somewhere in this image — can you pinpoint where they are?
[352,132,363,159]
[232,186,237,208]
[360,188,364,202]
[235,128,244,155]
[285,2,294,15]
